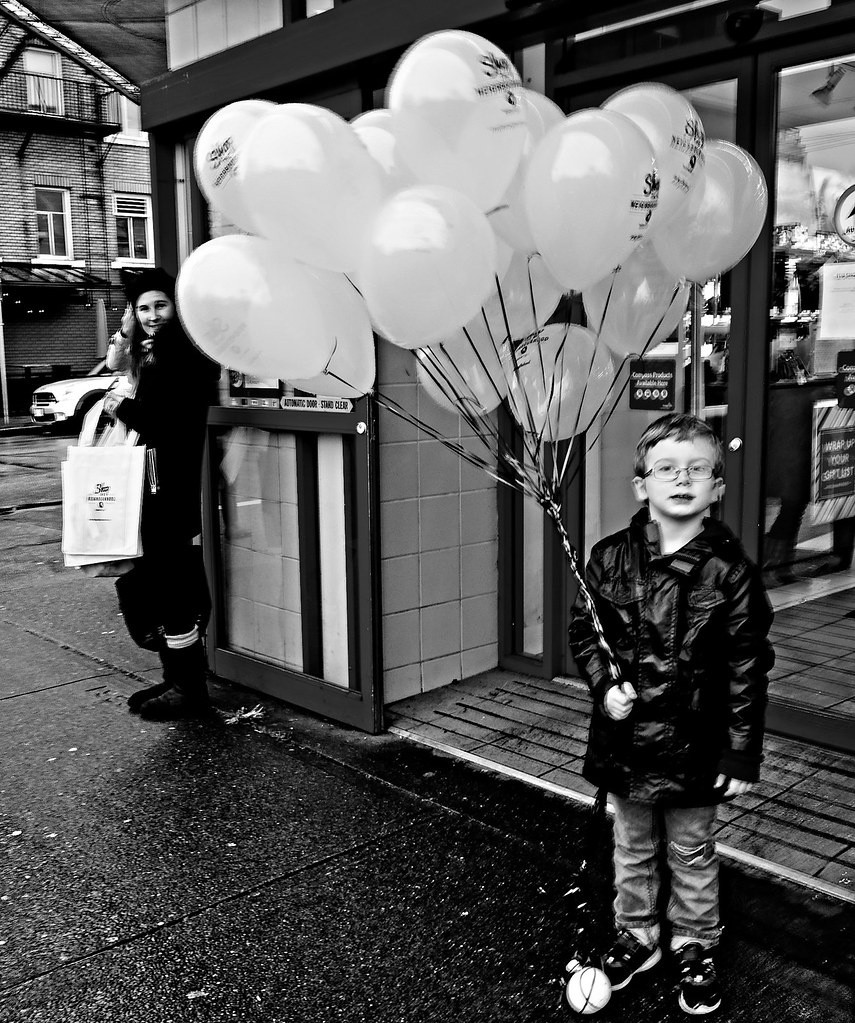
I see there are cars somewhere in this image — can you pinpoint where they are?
[30,358,127,436]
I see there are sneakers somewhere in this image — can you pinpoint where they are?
[601,931,662,991]
[673,940,723,1015]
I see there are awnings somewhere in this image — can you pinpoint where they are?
[0,265,111,288]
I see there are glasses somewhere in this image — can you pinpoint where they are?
[641,457,716,483]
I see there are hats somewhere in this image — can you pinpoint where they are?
[125,267,176,304]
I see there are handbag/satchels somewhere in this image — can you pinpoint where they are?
[60,397,147,568]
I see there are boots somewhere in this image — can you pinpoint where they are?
[139,636,213,722]
[127,636,176,709]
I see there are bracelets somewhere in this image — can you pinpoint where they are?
[120,329,128,338]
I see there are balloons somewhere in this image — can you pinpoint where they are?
[525,106,660,294]
[602,82,706,233]
[285,272,377,398]
[175,234,334,380]
[195,99,273,230]
[581,239,690,357]
[506,323,615,442]
[352,29,567,417]
[648,139,767,285]
[238,102,387,272]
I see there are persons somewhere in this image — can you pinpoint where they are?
[103,269,221,722]
[570,413,776,1016]
